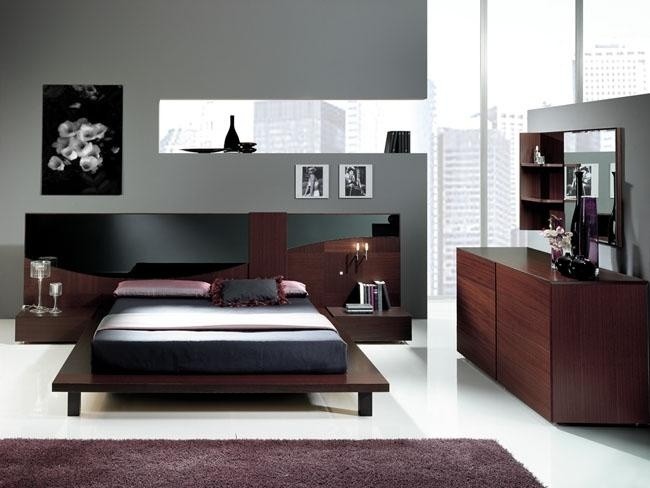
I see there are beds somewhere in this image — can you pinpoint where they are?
[52,273,390,417]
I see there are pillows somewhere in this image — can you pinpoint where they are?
[113,275,308,307]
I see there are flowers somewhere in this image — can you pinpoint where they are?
[543,225,574,246]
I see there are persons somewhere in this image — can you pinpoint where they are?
[579,166,592,196]
[300,166,323,198]
[343,166,367,197]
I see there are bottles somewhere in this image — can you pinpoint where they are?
[224,115,241,152]
[571,170,599,278]
[534,146,541,163]
[555,252,596,280]
[608,171,617,243]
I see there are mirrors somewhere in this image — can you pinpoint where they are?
[563,127,626,247]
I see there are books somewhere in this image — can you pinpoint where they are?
[383,130,411,154]
[344,279,392,315]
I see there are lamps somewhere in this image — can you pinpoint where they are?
[30,261,62,314]
[346,242,369,268]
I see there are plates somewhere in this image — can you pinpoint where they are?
[180,147,230,153]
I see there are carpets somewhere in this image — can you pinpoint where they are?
[0,439,543,486]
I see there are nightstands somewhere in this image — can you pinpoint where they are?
[15,302,92,342]
[327,302,412,344]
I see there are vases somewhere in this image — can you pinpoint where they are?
[548,241,565,269]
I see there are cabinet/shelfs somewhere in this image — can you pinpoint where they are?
[456,246,649,426]
[519,131,564,232]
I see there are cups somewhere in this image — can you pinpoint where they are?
[536,156,545,164]
